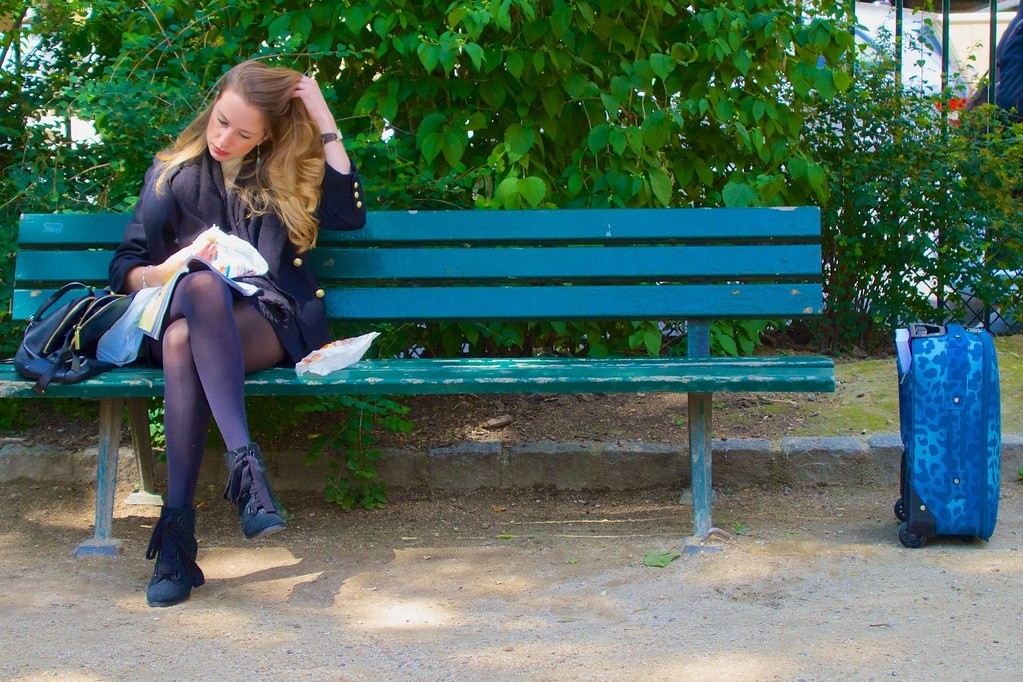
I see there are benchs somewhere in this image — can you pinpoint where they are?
[0,207,835,557]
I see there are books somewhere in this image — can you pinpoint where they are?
[138,255,259,340]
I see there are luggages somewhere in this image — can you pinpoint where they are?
[895,322,1001,549]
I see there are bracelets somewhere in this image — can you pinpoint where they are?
[142,265,153,288]
[320,129,343,147]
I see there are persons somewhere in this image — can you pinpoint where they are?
[109,61,366,607]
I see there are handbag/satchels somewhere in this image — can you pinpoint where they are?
[14,282,139,393]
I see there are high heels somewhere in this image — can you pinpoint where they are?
[223,442,287,539]
[145,506,205,606]
